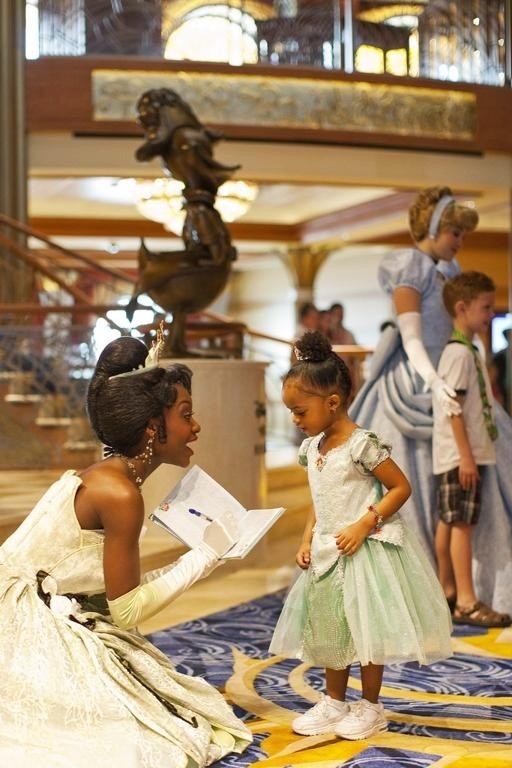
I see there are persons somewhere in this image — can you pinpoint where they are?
[267,330,456,739]
[432,271,511,626]
[1,336,254,768]
[294,302,356,344]
[346,183,512,628]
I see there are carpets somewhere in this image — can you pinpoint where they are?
[141,584,511,767]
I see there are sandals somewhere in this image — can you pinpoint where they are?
[453,600,511,628]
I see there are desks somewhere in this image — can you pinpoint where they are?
[255,14,411,76]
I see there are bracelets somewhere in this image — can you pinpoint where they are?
[366,504,383,535]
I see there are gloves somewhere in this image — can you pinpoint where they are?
[142,549,225,586]
[107,508,243,632]
[396,311,462,418]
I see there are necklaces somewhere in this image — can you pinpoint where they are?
[117,453,143,489]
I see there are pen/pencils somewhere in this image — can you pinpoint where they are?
[189,508,214,522]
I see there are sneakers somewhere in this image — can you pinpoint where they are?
[290,695,350,737]
[336,696,390,741]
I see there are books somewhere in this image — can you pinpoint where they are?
[150,463,287,560]
